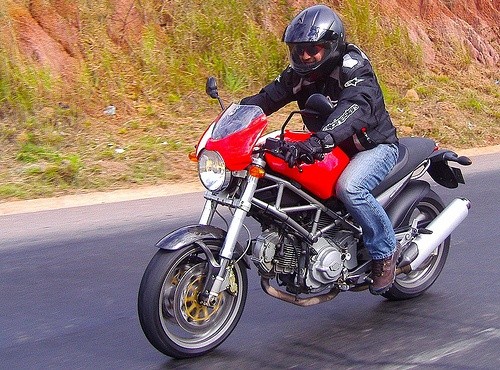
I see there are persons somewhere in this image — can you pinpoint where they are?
[211,6,402,296]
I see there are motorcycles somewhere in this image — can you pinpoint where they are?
[138,76,472,361]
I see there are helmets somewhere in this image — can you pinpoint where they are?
[284,5,345,78]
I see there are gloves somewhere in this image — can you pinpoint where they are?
[284,138,323,168]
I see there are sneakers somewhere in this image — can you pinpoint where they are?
[369,241,401,295]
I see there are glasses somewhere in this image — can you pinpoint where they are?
[296,45,325,56]
